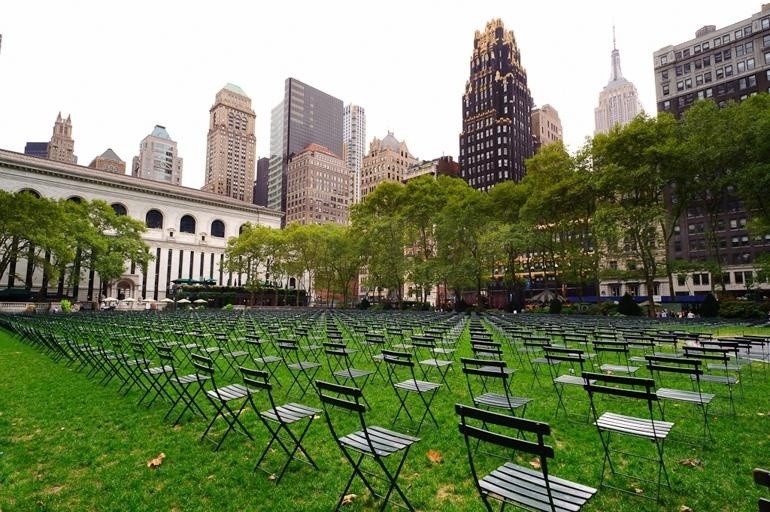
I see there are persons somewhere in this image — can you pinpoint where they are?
[654,307,697,320]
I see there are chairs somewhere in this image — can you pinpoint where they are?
[3,306,769,510]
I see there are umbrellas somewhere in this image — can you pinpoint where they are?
[100,295,208,311]
